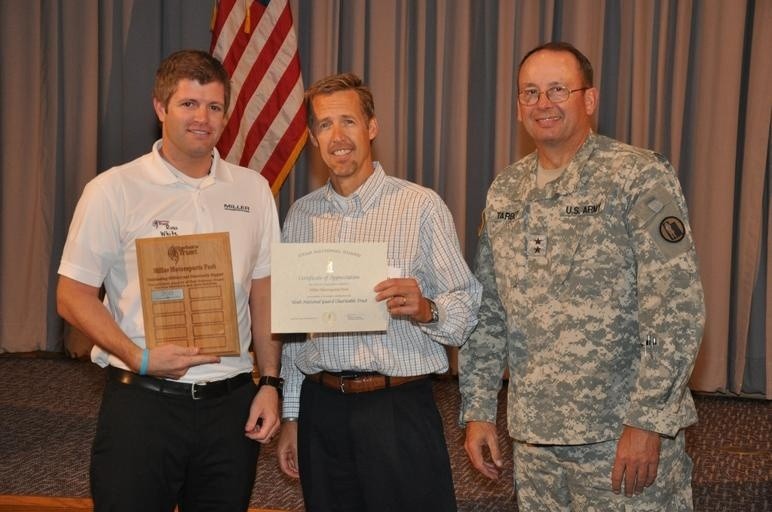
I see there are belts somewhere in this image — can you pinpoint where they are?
[308,372,429,396]
[104,365,253,400]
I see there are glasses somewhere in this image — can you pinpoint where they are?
[519,87,587,105]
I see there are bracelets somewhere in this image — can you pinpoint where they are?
[138,347,150,378]
[274,412,299,423]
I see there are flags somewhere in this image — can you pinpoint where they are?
[209,0,308,198]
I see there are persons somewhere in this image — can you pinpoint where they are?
[459,43,707,512]
[57,50,283,512]
[278,73,484,512]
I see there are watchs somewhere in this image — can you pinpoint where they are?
[259,375,284,391]
[428,300,438,322]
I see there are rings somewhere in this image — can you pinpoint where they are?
[403,297,407,305]
